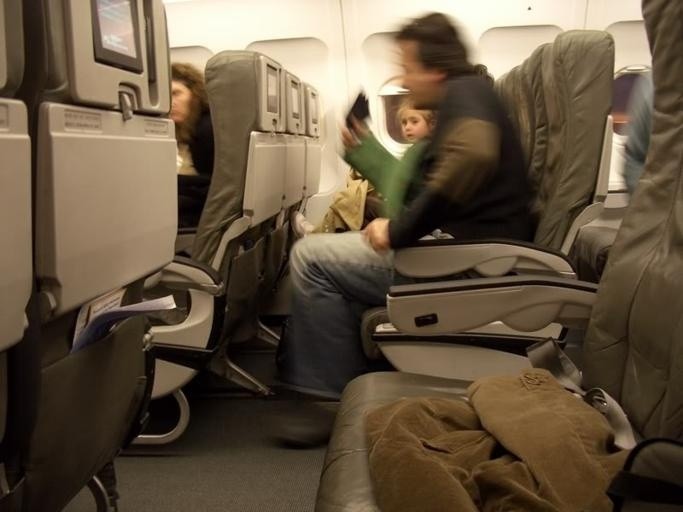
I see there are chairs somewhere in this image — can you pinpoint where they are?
[116,48,322,457]
[0,2,181,511]
[355,29,617,384]
[311,10,681,508]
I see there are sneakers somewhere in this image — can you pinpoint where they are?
[290,211,314,236]
[268,410,334,448]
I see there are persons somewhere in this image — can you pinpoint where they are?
[277,12,540,450]
[166,62,214,228]
